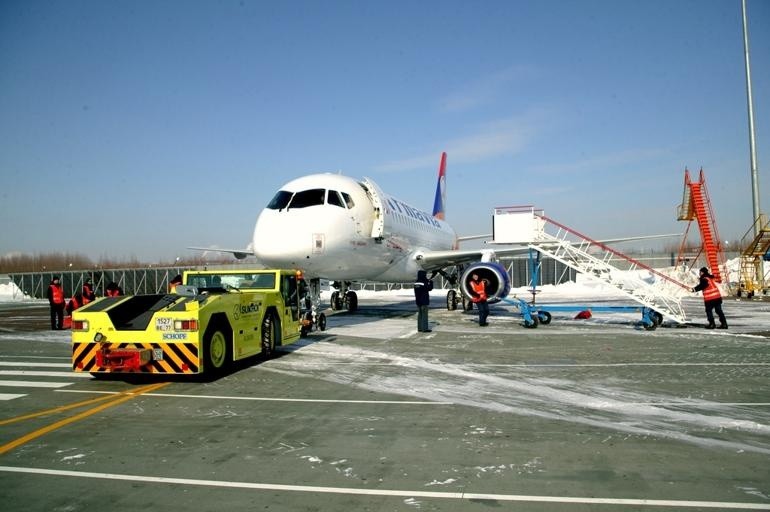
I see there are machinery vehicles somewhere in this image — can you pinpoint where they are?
[71,269,301,380]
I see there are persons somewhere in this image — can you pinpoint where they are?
[414,270,433,332]
[104,282,123,296]
[467,271,490,326]
[168,275,182,294]
[688,267,728,329]
[47,277,96,330]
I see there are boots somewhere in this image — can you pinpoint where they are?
[705,324,728,329]
[418,329,432,332]
[479,322,488,326]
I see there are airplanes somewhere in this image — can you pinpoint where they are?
[185,170,683,331]
